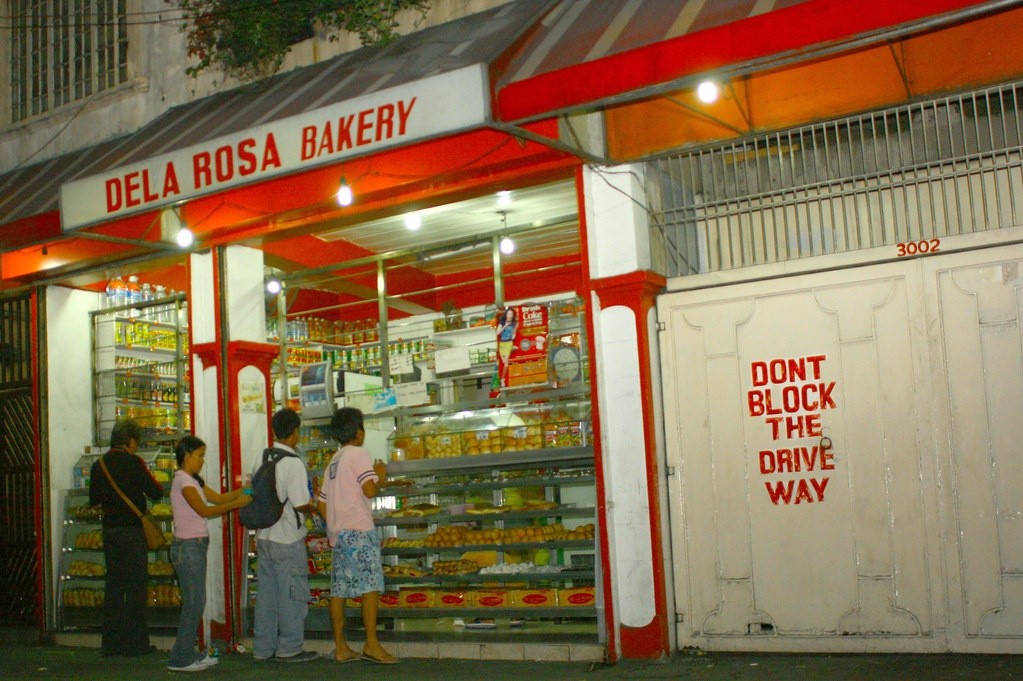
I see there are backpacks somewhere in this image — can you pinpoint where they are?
[237,448,301,529]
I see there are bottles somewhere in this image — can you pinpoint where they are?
[116,377,191,403]
[267,316,378,345]
[302,447,336,469]
[308,475,324,508]
[303,511,320,531]
[105,275,189,332]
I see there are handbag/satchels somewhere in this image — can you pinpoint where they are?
[140,510,167,550]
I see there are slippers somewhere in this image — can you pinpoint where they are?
[360,651,401,664]
[334,652,363,663]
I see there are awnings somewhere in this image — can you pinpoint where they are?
[0,0,1023,284]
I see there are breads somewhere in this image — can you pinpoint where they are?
[303,415,597,608]
[62,500,181,607]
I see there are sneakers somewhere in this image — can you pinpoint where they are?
[202,655,219,666]
[166,659,209,671]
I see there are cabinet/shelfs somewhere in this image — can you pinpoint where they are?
[246,312,599,640]
[54,293,188,627]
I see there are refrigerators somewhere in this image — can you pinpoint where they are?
[294,363,398,593]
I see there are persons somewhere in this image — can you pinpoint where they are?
[496,307,518,386]
[89,417,164,656]
[317,406,401,664]
[166,435,254,671]
[254,407,321,662]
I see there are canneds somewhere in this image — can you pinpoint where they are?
[114,320,191,430]
[288,340,426,368]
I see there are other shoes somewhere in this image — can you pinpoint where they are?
[101,644,157,657]
[276,648,321,662]
[254,652,282,662]
